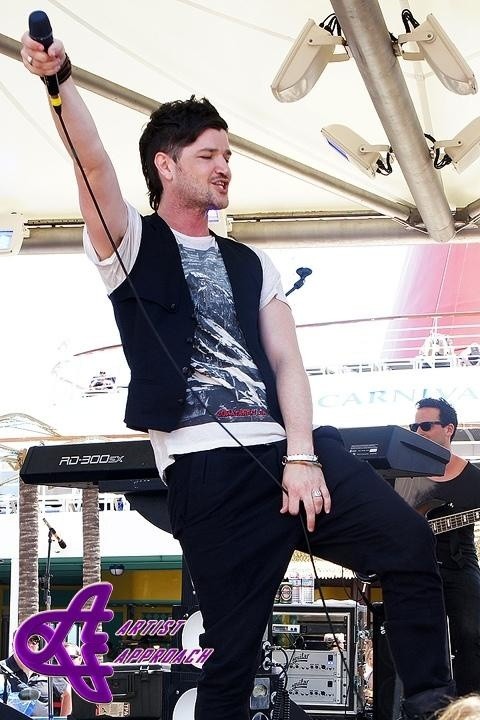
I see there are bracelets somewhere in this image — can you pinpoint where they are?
[281,451,323,468]
[39,52,73,89]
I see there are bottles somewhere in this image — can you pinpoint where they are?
[288,564,316,605]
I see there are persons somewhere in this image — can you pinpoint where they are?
[393,394,479,700]
[0,626,75,720]
[52,641,84,716]
[322,630,375,706]
[19,25,479,717]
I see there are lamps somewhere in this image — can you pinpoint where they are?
[388,9,480,174]
[109,564,124,576]
[270,12,394,179]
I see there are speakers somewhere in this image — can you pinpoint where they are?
[161,552,273,720]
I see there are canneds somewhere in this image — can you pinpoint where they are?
[280,583,293,604]
[274,584,281,604]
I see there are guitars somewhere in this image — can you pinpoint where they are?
[355,499,479,581]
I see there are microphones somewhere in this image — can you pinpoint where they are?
[42,518,67,549]
[28,10,62,116]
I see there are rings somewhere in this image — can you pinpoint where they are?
[311,490,322,498]
[25,53,35,67]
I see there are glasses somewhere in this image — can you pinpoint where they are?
[409,421,443,432]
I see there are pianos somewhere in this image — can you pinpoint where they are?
[20,425,451,494]
